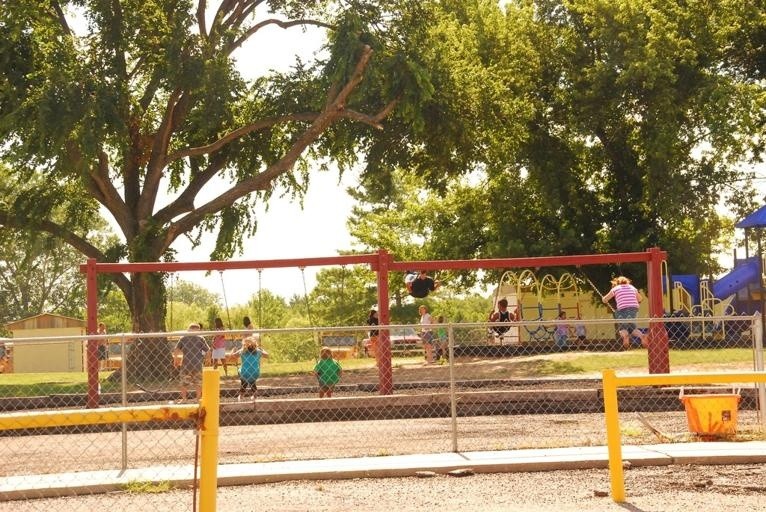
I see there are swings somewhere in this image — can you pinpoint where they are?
[131,272,174,390]
[217,268,263,382]
[403,268,436,297]
[298,265,346,388]
[577,263,638,330]
[486,268,515,334]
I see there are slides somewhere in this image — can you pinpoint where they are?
[632,257,760,344]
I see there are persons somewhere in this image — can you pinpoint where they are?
[173,323,210,405]
[551,311,569,354]
[210,316,228,377]
[366,308,379,369]
[599,275,647,349]
[435,314,448,363]
[96,322,108,372]
[400,270,441,300]
[487,298,522,339]
[312,346,342,398]
[570,313,595,352]
[417,304,435,366]
[229,336,269,399]
[230,315,260,355]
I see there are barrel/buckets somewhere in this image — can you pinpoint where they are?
[678,393,742,434]
